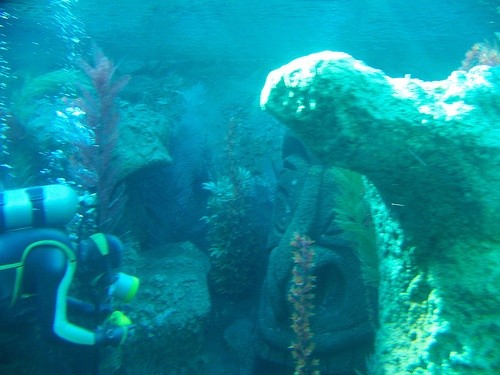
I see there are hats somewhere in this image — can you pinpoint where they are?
[88,232,123,274]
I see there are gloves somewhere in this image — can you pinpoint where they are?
[105,325,135,348]
[103,302,129,317]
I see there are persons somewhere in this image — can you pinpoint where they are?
[0,229,143,350]
